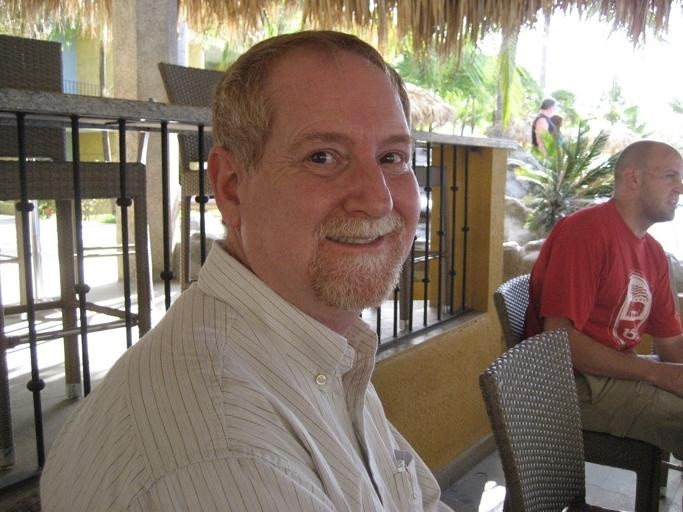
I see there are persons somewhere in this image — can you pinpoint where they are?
[524,139,683,461]
[550,114,566,146]
[532,97,556,156]
[35,24,458,511]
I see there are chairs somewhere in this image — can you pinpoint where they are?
[494,274,660,512]
[387,165,452,331]
[478,328,624,512]
[1,35,151,470]
[157,62,225,293]
